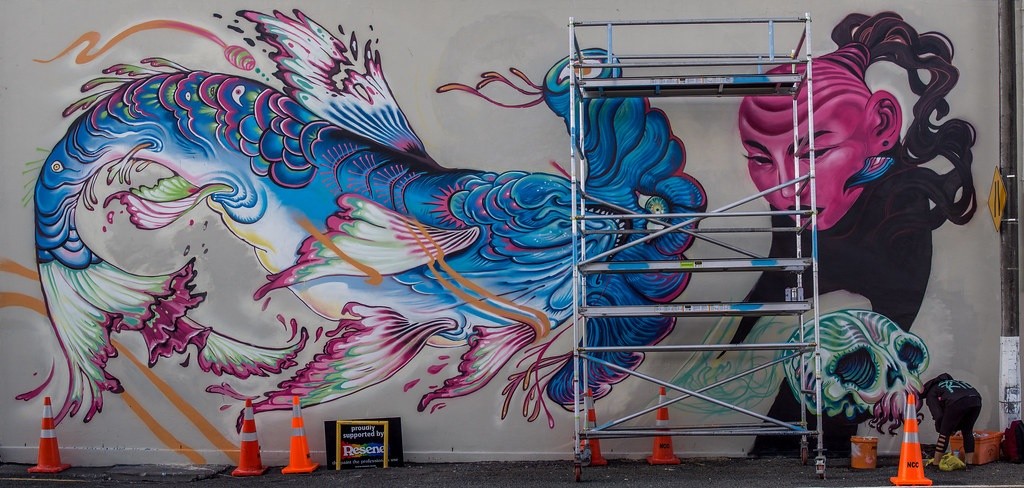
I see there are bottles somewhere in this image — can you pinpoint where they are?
[954,448,960,458]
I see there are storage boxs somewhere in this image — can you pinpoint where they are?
[948,430,1005,465]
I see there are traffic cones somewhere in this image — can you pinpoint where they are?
[890,393,933,486]
[232,397,270,477]
[646,386,681,465]
[581,387,608,466]
[281,396,319,473]
[27,396,72,474]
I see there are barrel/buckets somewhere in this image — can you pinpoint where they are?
[850,436,878,469]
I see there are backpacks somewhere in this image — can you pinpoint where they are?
[1000,421,1024,463]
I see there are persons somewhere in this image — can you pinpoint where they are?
[921,374,982,471]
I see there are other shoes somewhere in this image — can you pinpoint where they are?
[966,463,972,471]
[930,464,940,472]
